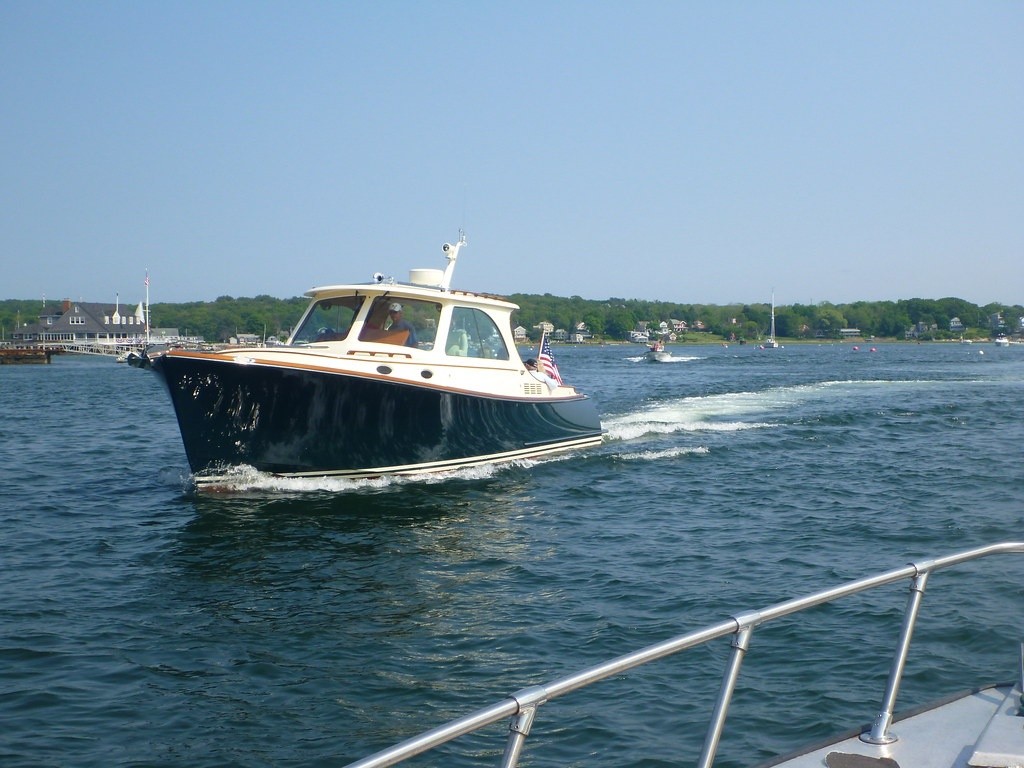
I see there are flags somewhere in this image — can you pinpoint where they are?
[134,302,146,323]
[143,273,150,287]
[539,334,563,386]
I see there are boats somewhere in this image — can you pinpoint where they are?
[643,341,672,361]
[129,227,603,502]
[995,337,1009,347]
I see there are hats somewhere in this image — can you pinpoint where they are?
[389,303,402,312]
[523,359,538,368]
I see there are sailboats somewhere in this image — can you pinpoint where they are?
[762,290,779,348]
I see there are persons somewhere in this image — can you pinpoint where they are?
[388,303,418,348]
[445,316,468,356]
[523,359,558,388]
